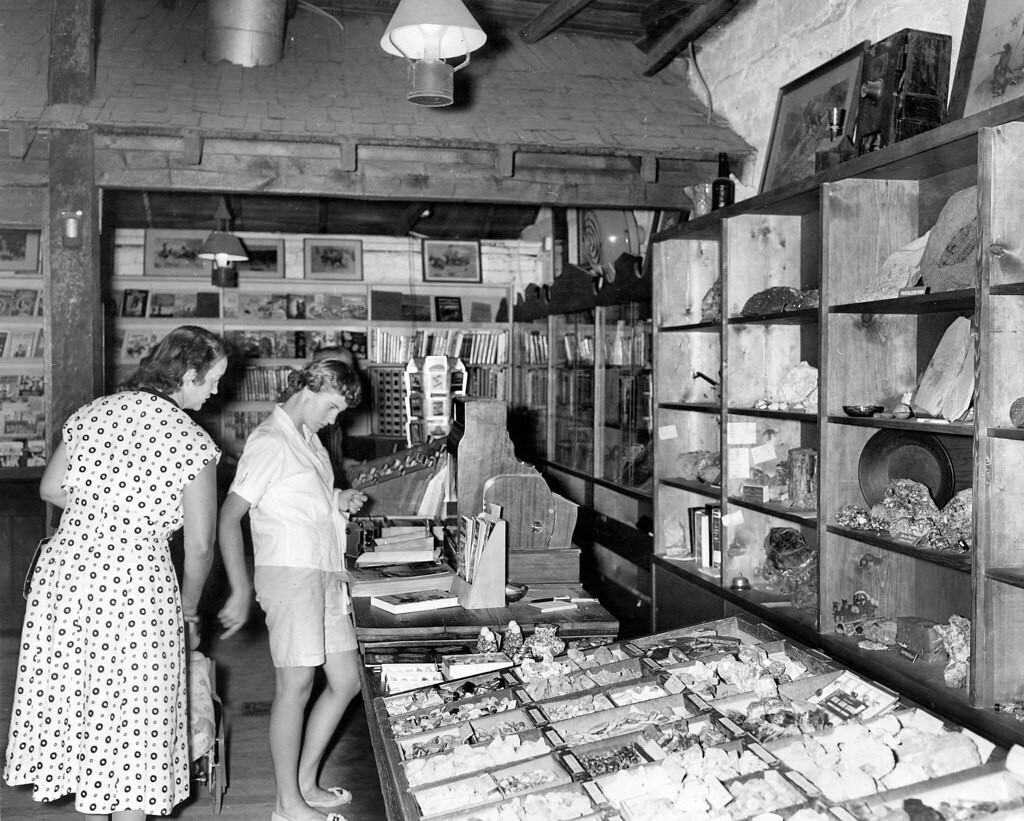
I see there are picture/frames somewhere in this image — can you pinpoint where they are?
[233,238,284,277]
[0,228,41,273]
[421,237,483,283]
[304,238,363,281]
[946,0,1024,122]
[759,40,870,194]
[144,228,213,276]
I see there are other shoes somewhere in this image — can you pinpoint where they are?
[271,811,348,821]
[305,787,352,808]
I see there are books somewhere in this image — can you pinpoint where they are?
[224,365,292,442]
[371,329,508,398]
[522,330,650,484]
[687,502,722,569]
[348,515,496,615]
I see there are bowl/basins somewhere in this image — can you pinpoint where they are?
[842,405,885,417]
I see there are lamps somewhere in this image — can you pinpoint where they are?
[197,195,249,287]
[380,0,487,108]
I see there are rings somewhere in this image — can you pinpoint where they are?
[357,508,360,511]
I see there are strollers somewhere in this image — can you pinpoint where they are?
[186,643,231,816]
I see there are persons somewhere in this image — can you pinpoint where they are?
[3,325,229,821]
[314,346,368,488]
[218,360,368,820]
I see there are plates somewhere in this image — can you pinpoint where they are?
[858,429,954,509]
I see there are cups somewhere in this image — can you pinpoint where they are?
[787,449,818,510]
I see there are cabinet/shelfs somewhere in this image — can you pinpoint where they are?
[0,97,1024,821]
